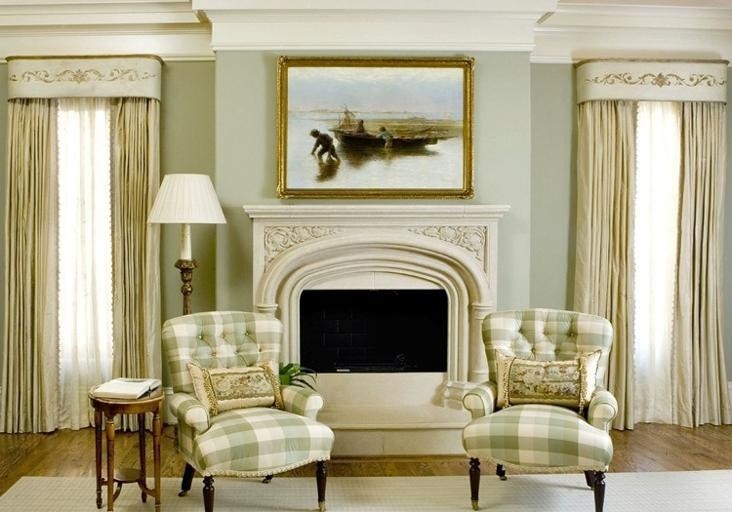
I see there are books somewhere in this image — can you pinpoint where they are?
[93,377,162,400]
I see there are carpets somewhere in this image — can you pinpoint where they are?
[0,469,731,511]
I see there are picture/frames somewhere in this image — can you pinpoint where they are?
[277,55,473,200]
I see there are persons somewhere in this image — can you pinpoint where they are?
[376,126,394,149]
[354,119,369,136]
[309,128,340,164]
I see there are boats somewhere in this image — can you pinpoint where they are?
[329,126,438,151]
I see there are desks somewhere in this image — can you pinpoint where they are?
[89,383,165,512]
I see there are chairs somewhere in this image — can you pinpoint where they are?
[462,308,618,511]
[162,311,335,512]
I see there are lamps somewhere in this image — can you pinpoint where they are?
[147,173,228,315]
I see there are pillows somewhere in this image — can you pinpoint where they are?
[185,360,286,417]
[494,350,602,409]
